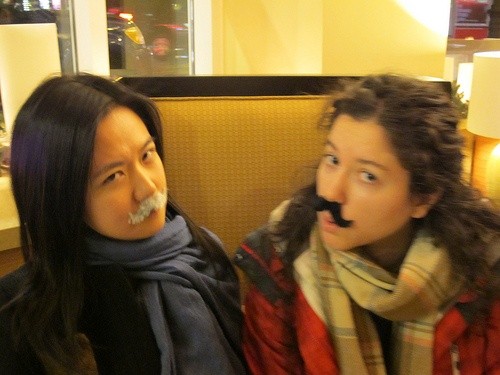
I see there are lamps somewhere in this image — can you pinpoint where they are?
[464,48,500,217]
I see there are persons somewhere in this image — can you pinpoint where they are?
[0,73,254,375]
[231,74,500,375]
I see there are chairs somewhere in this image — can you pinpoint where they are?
[106,69,455,312]
